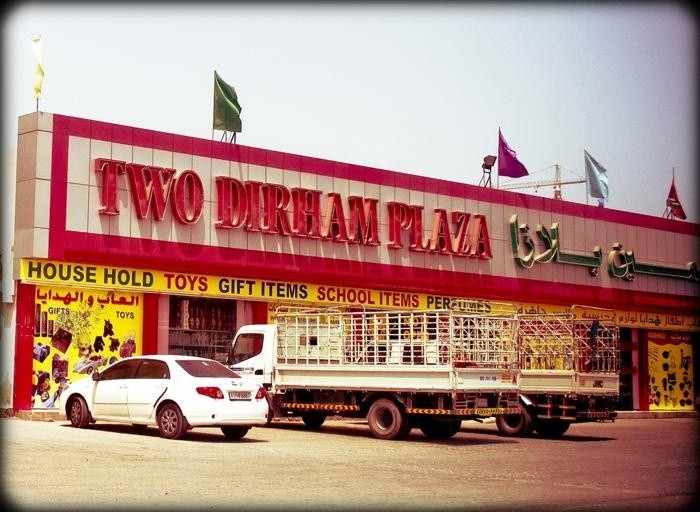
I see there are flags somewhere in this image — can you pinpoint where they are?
[211,70,244,135]
[666,167,692,221]
[496,127,531,179]
[583,149,611,202]
[31,33,46,105]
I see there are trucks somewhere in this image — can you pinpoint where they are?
[357,305,623,438]
[225,303,522,440]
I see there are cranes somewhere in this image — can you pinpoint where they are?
[490,162,586,200]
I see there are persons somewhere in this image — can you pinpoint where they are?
[32,371,66,409]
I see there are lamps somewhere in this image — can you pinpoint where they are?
[477,155,497,188]
[661,198,679,219]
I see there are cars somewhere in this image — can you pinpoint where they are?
[55,352,271,440]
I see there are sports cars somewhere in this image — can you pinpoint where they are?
[72,355,109,376]
